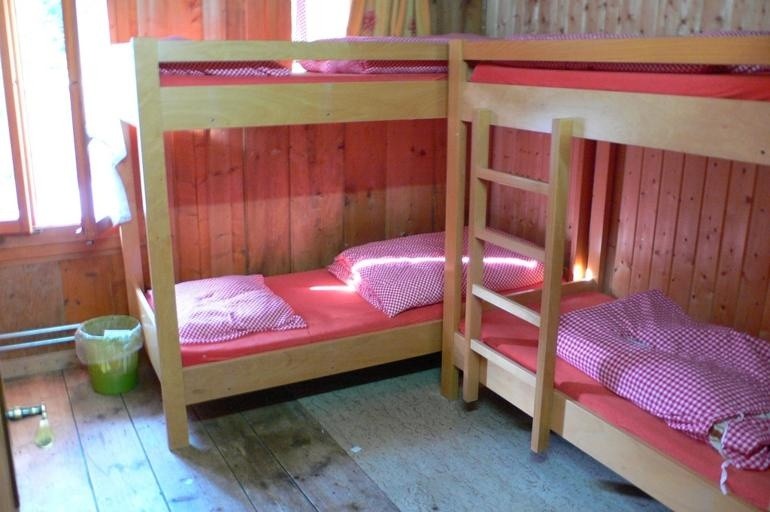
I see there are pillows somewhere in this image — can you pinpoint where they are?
[146,274,307,345]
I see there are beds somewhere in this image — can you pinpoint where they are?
[111,33,616,450]
[439,32,770,511]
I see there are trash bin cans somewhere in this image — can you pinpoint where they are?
[80,315,142,395]
